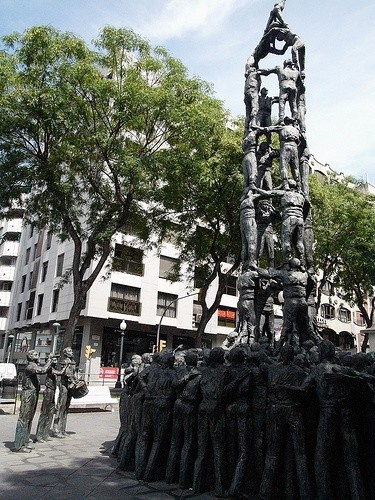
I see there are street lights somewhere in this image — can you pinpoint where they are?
[115,319,127,389]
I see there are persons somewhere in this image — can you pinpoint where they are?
[13,350,52,453]
[51,347,75,439]
[36,354,68,443]
[112,0,372,499]
